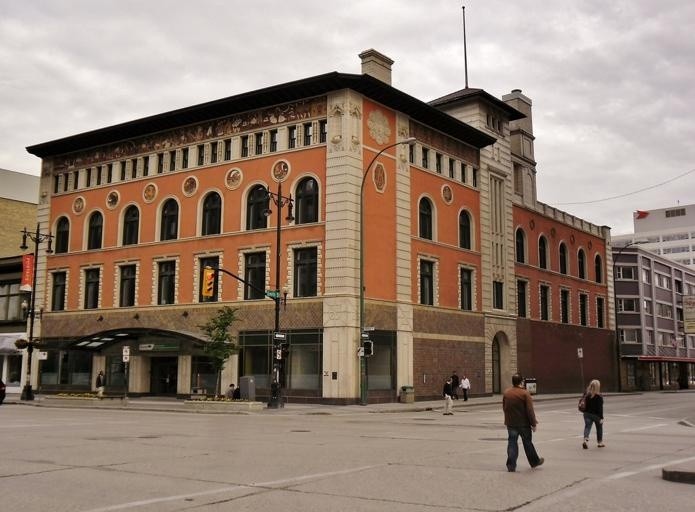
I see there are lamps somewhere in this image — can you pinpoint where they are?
[97,315,103,322]
[181,310,188,319]
[133,313,139,320]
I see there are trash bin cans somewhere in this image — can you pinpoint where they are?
[400,386,414,403]
[523,376,537,395]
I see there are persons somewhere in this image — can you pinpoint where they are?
[233,388,239,399]
[451,371,459,400]
[503,373,544,472]
[225,384,234,398]
[443,378,453,415]
[459,375,470,401]
[95,371,106,397]
[582,380,605,449]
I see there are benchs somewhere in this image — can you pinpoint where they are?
[96,394,125,400]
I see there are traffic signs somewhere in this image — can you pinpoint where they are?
[267,290,280,299]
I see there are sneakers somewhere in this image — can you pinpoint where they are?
[583,441,588,450]
[508,467,516,473]
[532,457,545,468]
[597,442,606,448]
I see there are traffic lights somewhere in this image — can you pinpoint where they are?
[361,339,375,358]
[277,342,291,366]
[201,267,218,299]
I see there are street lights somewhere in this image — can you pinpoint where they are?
[255,178,302,409]
[354,129,422,410]
[13,224,60,406]
[608,234,653,396]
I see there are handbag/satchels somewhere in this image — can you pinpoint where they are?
[578,392,588,412]
